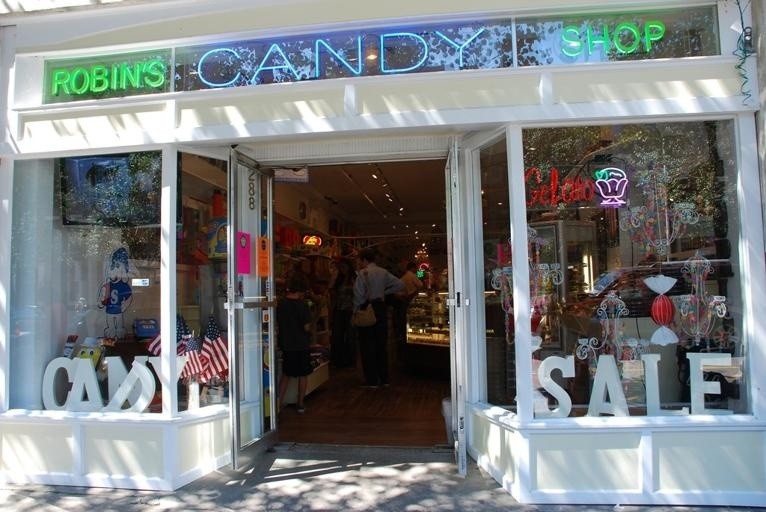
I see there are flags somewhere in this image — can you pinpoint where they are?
[196,309,230,388]
[143,310,204,380]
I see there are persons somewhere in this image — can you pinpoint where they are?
[353,249,407,389]
[276,271,318,414]
[330,255,355,368]
[393,261,424,304]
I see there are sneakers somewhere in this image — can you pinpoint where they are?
[375,379,390,385]
[360,383,378,388]
[296,405,305,415]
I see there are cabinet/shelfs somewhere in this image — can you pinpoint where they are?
[394,287,499,379]
[180,164,396,418]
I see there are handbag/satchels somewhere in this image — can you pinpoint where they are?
[353,305,376,326]
